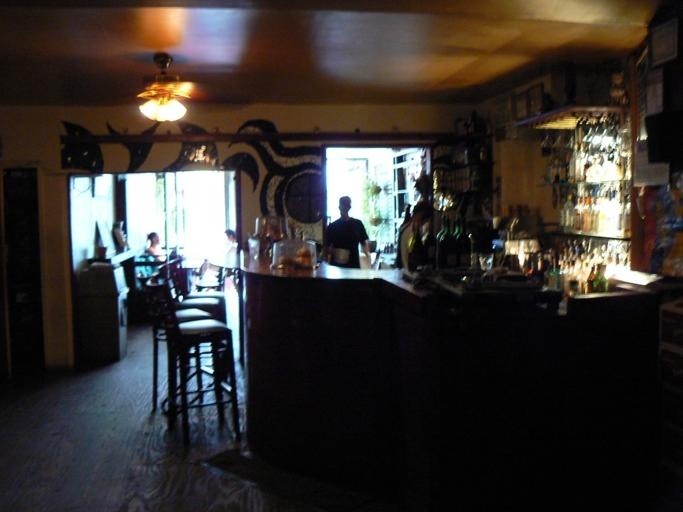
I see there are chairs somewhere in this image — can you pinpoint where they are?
[143,257,242,445]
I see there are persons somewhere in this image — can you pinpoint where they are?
[143,232,168,267]
[319,194,369,272]
[223,229,236,244]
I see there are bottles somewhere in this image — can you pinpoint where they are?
[541,116,629,237]
[402,204,612,296]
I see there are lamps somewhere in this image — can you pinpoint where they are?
[135,50,196,125]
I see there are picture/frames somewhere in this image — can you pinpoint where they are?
[514,80,545,120]
[647,14,679,68]
